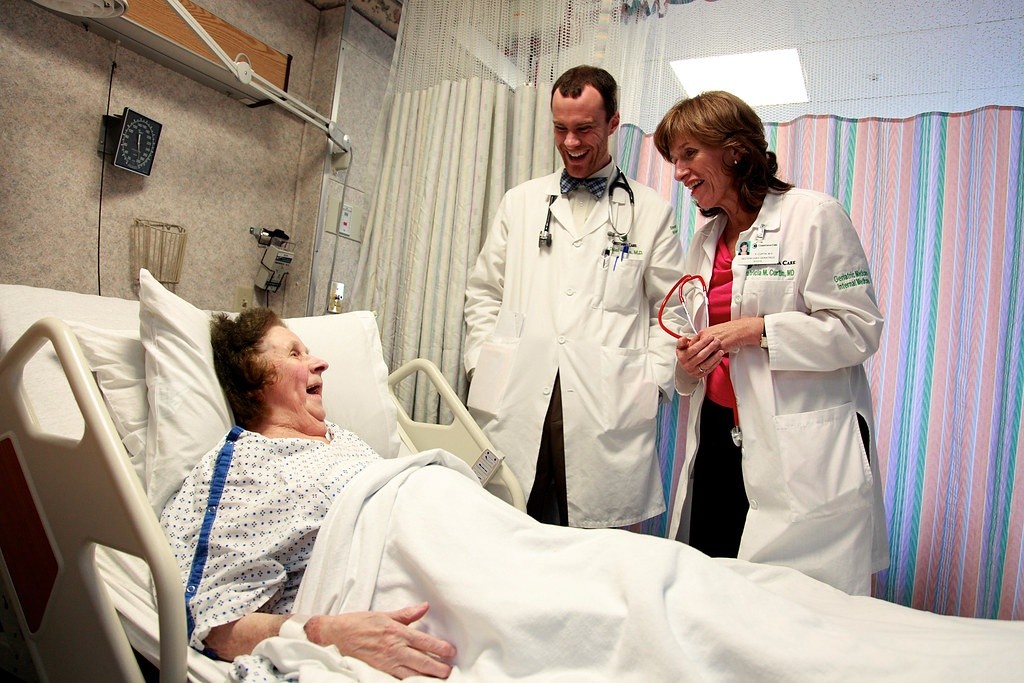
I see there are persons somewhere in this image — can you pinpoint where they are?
[654,90,895,601]
[152,307,457,681]
[463,65,685,533]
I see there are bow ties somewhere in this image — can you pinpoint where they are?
[560,167,608,199]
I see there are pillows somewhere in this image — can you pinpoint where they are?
[140,268,400,514]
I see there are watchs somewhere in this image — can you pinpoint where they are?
[760,325,769,350]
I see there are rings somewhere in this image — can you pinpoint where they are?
[698,365,705,373]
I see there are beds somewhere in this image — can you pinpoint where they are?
[0,284,1024,683]
